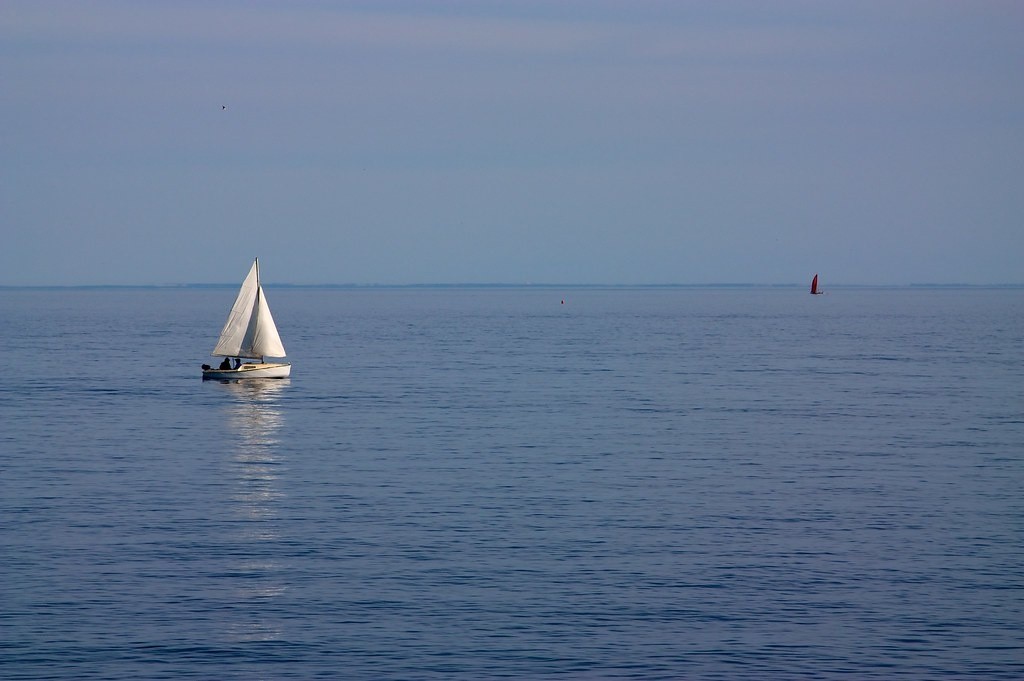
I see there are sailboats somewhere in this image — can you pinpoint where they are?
[810,274,824,295]
[202,257,292,379]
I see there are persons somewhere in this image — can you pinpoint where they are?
[219,358,242,370]
